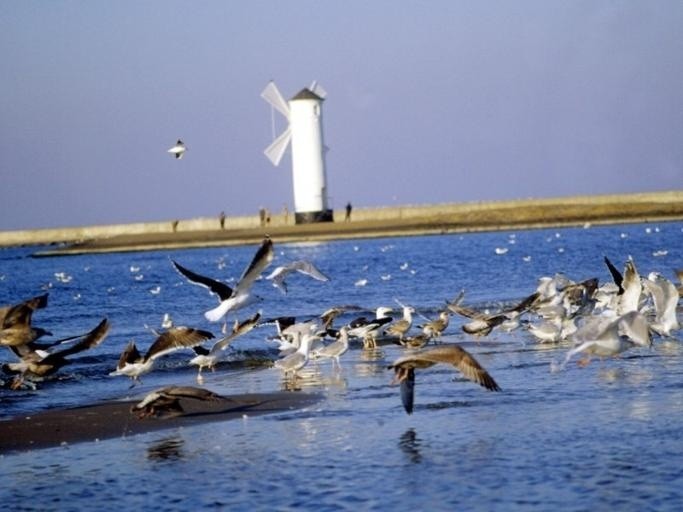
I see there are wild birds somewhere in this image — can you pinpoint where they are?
[0,235,683,420]
[167,137,188,159]
